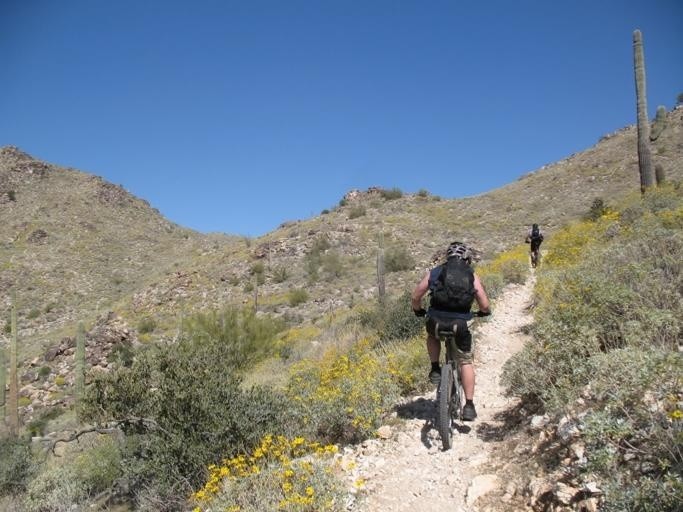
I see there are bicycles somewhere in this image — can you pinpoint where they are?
[526,240,542,268]
[417,309,491,450]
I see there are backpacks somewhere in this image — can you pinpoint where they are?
[430,263,476,315]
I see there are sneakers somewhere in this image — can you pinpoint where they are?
[428,368,441,385]
[461,404,479,422]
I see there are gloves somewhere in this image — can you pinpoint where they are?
[413,306,427,317]
[475,309,492,318]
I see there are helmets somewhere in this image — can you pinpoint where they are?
[445,242,472,264]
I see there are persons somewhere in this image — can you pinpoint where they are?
[523,222,544,270]
[409,241,493,423]
